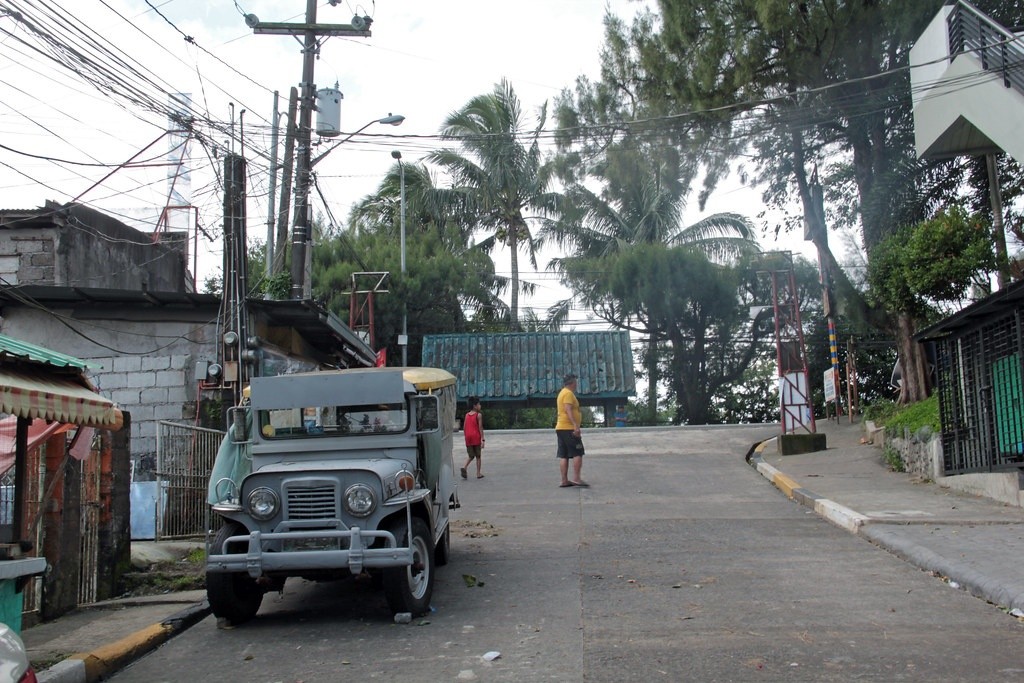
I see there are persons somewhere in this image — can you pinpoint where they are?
[555,374,589,488]
[460,396,485,479]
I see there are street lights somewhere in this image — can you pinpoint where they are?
[390,150,408,367]
[286,113,407,301]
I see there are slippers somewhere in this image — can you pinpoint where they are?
[477,475,484,478]
[572,481,590,487]
[558,481,575,487]
[460,468,467,478]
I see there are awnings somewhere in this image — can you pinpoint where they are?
[0,364,117,426]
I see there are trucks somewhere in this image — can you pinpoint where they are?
[201,363,460,625]
